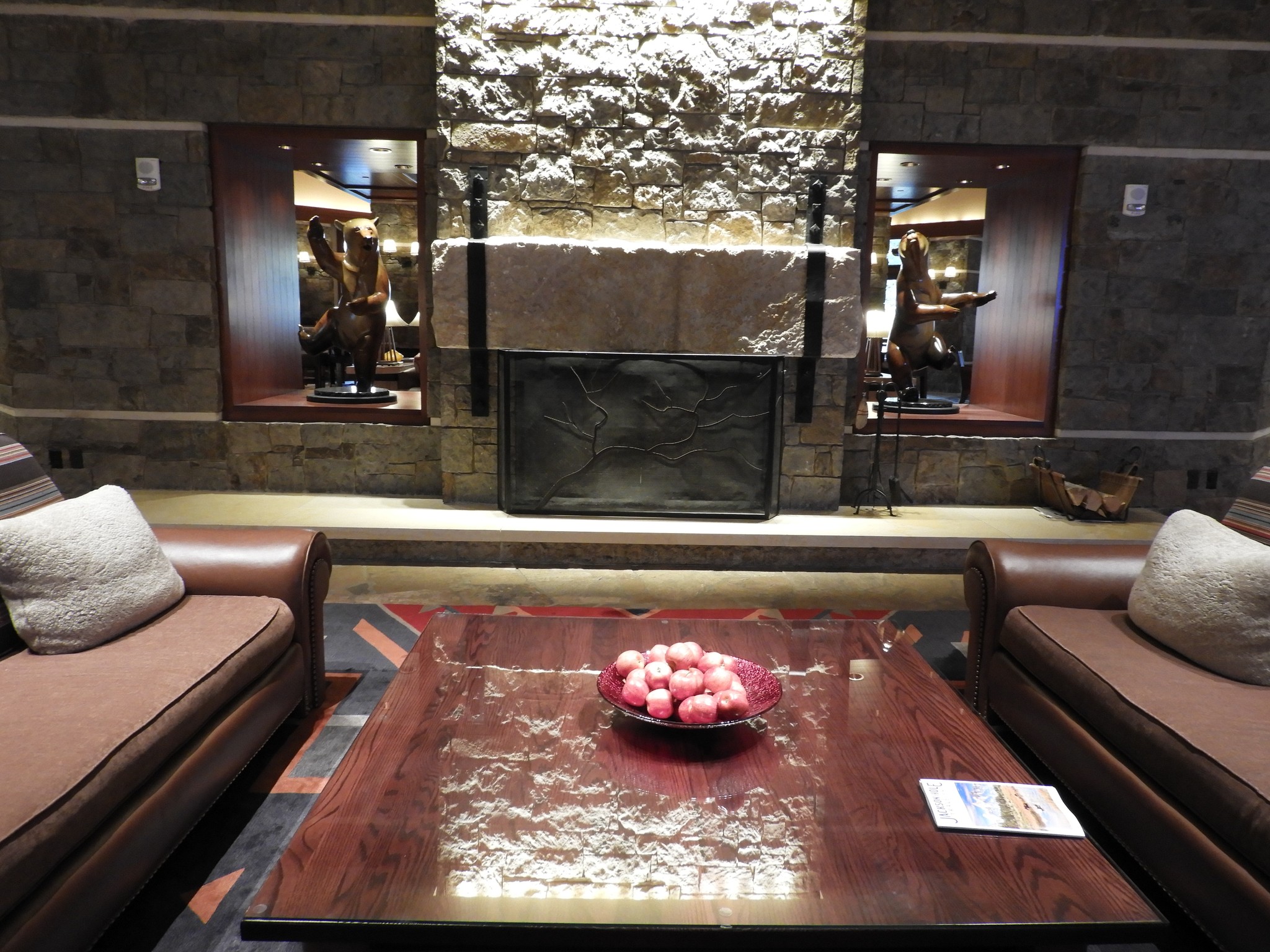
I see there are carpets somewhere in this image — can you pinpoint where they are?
[101,599,1170,952]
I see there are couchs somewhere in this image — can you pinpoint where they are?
[0,522,334,951]
[963,536,1270,952]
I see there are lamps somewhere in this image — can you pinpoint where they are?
[299,251,324,275]
[928,266,957,290]
[864,310,890,376]
[383,239,419,268]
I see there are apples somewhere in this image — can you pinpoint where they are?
[617,642,748,723]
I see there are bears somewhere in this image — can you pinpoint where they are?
[885,227,999,402]
[297,213,390,393]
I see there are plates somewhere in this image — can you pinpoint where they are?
[597,651,782,729]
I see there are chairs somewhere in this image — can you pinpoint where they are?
[303,326,420,391]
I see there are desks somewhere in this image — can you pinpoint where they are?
[345,362,414,390]
[239,604,1162,952]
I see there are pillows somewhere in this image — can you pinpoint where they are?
[1127,462,1270,690]
[0,430,187,657]
